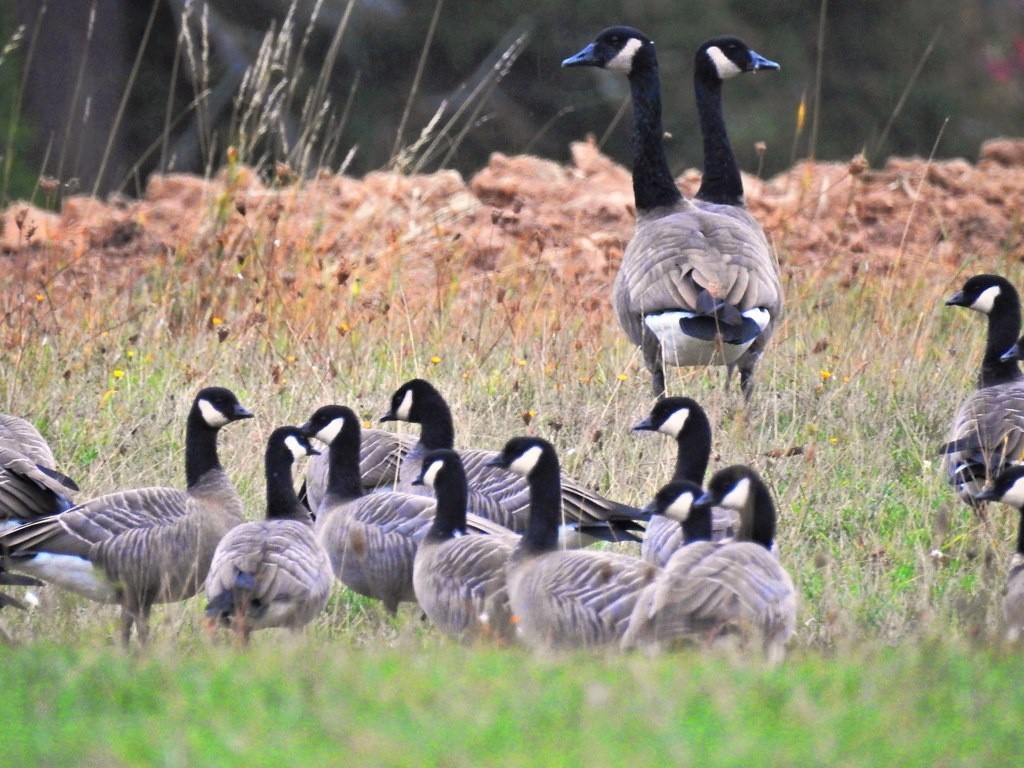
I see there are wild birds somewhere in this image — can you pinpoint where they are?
[942,275,1024,572]
[0,411,79,642]
[560,26,784,413]
[411,395,795,663]
[79,377,462,644]
